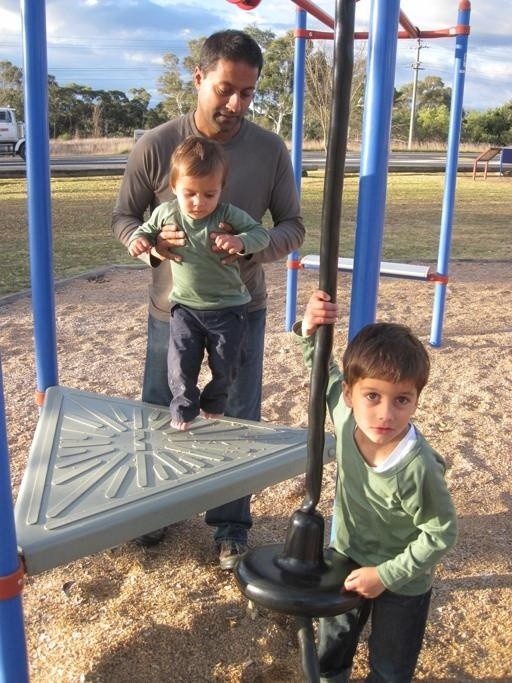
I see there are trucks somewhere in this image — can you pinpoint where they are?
[0,107,26,161]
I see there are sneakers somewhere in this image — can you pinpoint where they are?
[137,526,167,544]
[217,537,246,571]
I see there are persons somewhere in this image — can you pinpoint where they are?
[107,26,306,574]
[290,287,461,683]
[127,136,271,431]
